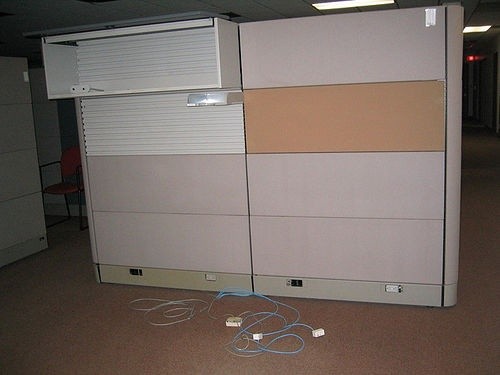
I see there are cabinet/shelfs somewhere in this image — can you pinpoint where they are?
[41,5,463,309]
[0,57,50,267]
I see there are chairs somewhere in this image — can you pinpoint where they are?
[39,147,88,232]
